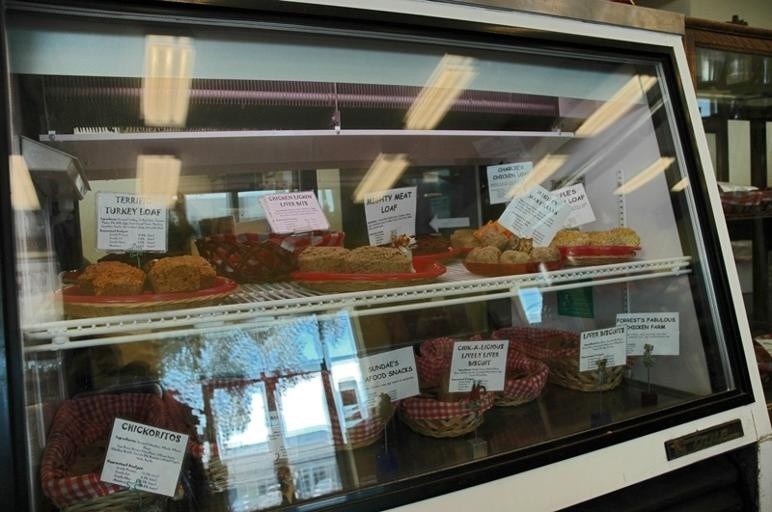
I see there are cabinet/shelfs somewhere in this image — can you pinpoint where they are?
[1,1,772,512]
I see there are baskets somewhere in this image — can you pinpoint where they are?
[399,336,550,439]
[193,228,346,284]
[491,325,625,392]
[39,369,398,510]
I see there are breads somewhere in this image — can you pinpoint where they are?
[147,254,217,295]
[77,260,145,299]
[451,221,640,266]
[298,245,349,274]
[342,246,413,275]
